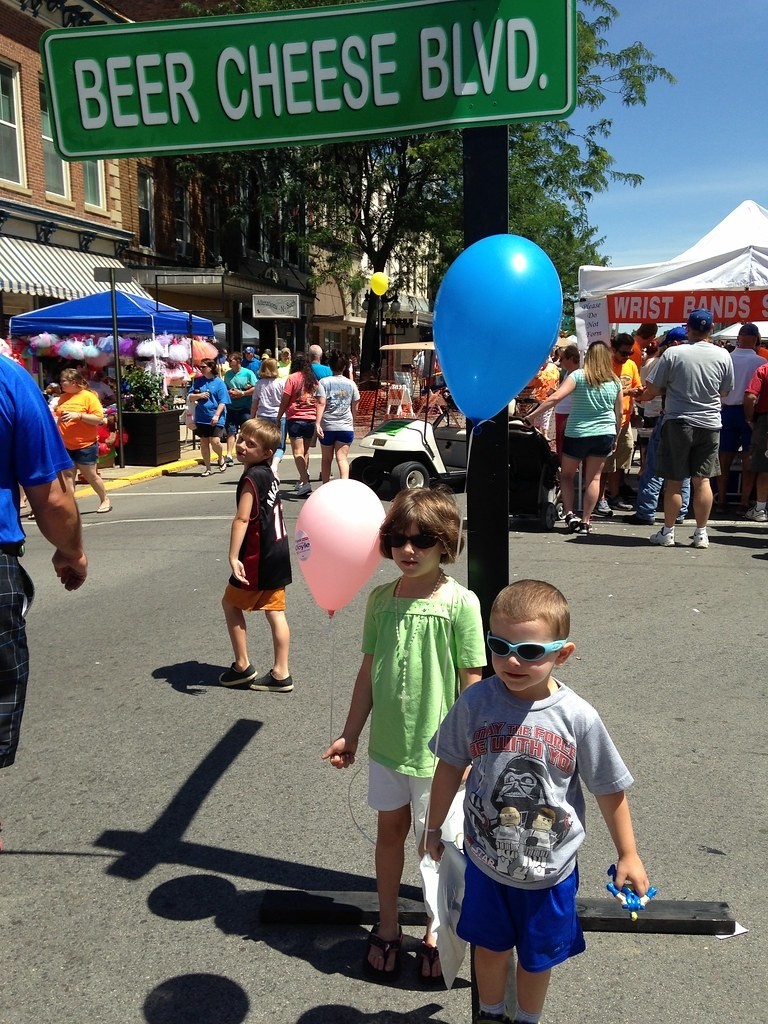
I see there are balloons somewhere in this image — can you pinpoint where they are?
[434,234,562,427]
[295,478,386,618]
[372,272,389,295]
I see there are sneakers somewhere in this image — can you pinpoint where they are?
[294,482,304,490]
[693,532,709,548]
[650,527,675,546]
[559,497,768,536]
[296,481,311,495]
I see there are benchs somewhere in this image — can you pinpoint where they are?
[433,427,468,469]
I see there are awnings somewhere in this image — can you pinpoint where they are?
[0,234,158,302]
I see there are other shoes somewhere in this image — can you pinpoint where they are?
[319,471,334,481]
[472,1010,535,1024]
[251,668,294,690]
[218,661,257,685]
[224,455,234,465]
[275,475,280,485]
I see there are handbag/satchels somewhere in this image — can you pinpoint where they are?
[186,393,197,430]
[421,838,468,991]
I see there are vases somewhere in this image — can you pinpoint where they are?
[118,409,186,470]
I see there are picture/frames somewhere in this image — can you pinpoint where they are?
[252,294,300,318]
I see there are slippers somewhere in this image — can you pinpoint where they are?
[367,921,402,973]
[420,933,445,982]
[218,459,225,471]
[202,470,211,477]
[96,505,113,513]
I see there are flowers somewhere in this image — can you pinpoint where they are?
[117,363,174,411]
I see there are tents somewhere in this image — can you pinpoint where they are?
[10,287,215,406]
[579,197,768,367]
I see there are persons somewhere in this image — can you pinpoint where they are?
[605,863,657,921]
[323,487,489,984]
[188,358,231,478]
[418,578,649,1023]
[212,338,361,497]
[743,362,768,521]
[0,352,87,772]
[218,417,294,691]
[635,308,734,549]
[19,367,116,520]
[523,341,624,535]
[515,323,768,521]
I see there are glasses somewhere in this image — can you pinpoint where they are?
[487,630,566,661]
[615,347,634,357]
[200,366,209,368]
[247,353,251,354]
[387,532,439,549]
[226,358,237,362]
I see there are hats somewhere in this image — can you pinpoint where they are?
[282,347,291,355]
[659,327,688,348]
[689,309,713,330]
[261,353,270,358]
[245,346,255,353]
